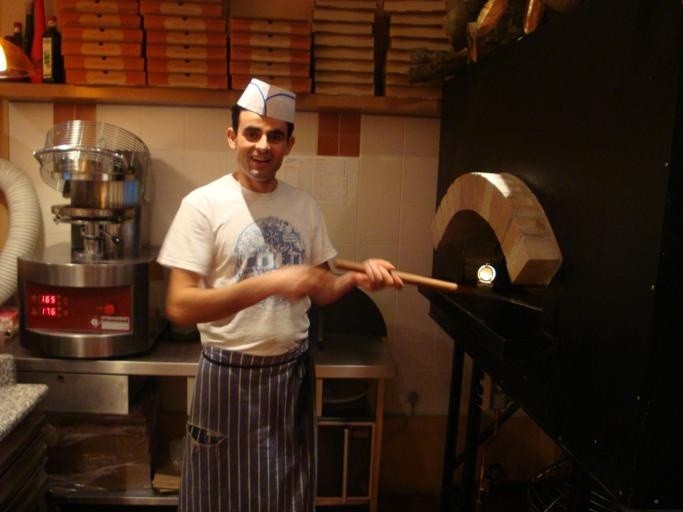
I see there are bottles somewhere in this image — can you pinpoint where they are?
[41,15,64,84]
[11,21,23,49]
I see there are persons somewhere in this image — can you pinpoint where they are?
[156,77,405,512]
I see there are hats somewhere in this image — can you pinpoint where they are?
[237,78,296,124]
